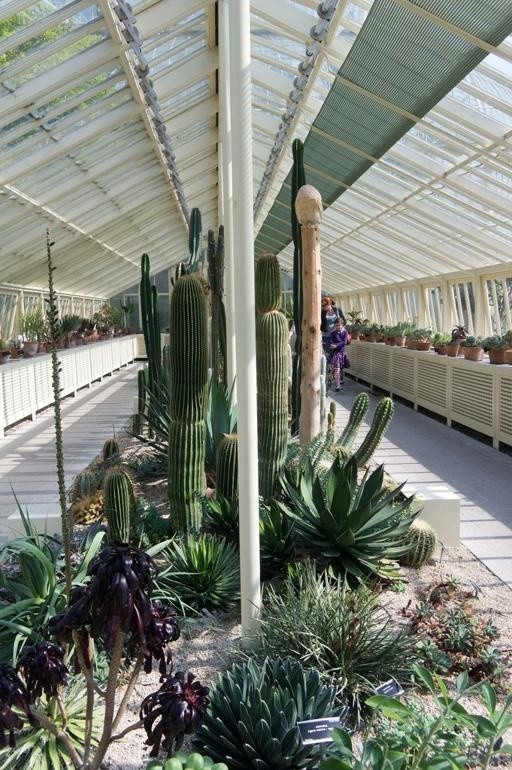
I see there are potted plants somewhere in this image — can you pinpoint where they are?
[346,308,512,364]
[0,297,136,364]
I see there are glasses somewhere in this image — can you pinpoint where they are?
[321,304,327,307]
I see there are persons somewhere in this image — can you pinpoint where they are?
[328,317,351,391]
[320,297,347,385]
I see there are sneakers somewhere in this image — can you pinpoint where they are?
[334,379,345,393]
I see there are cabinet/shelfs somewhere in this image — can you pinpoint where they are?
[0,332,170,439]
[342,337,512,451]
